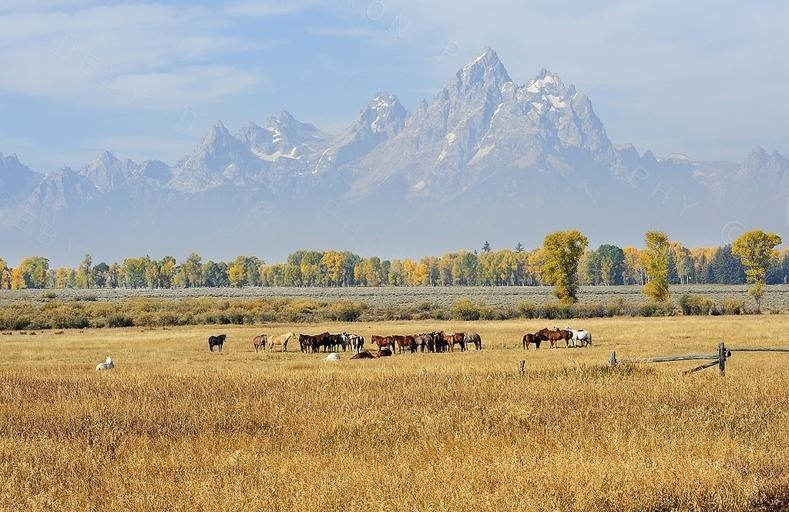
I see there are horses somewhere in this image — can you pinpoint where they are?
[96,354,115,371]
[324,352,341,360]
[298,331,365,353]
[351,352,372,359]
[522,325,592,350]
[371,331,481,357]
[267,330,297,353]
[253,334,267,353]
[207,334,227,352]
